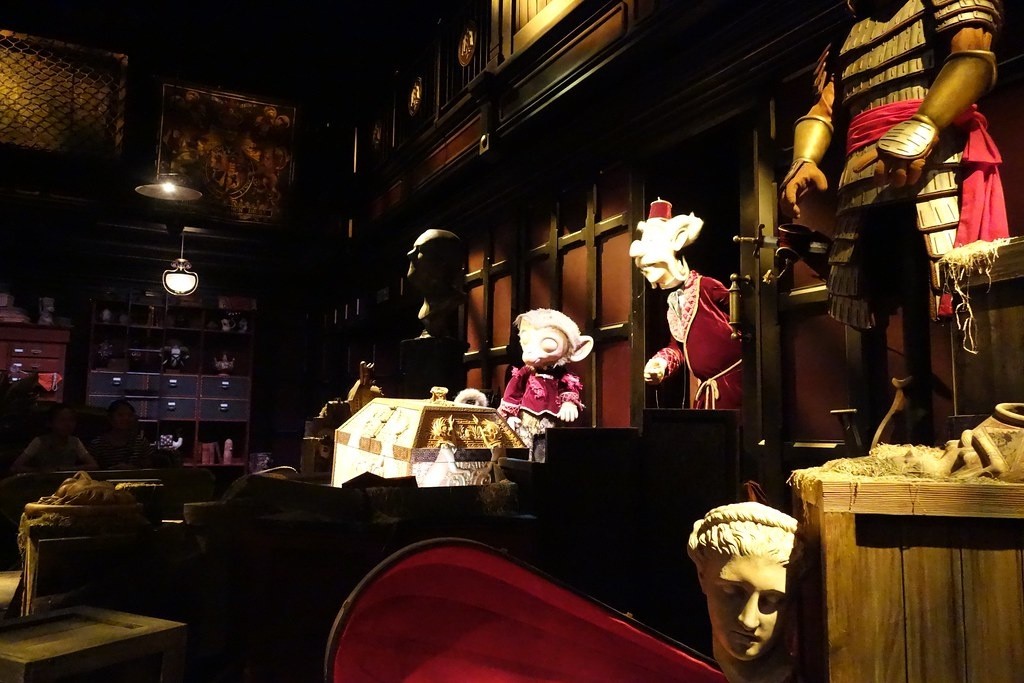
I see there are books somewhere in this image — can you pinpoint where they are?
[197,441,223,465]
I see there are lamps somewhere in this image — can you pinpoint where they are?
[134,166,203,201]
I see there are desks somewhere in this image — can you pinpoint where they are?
[0,570,189,683]
[0,468,217,600]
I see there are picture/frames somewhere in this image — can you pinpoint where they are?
[152,75,301,231]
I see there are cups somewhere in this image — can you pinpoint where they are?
[778,223,811,260]
[221,319,236,331]
[202,443,215,464]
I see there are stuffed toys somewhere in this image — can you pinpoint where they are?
[497,308,594,435]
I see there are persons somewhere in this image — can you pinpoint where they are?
[686,502,814,683]
[8,403,99,472]
[406,228,467,328]
[89,399,155,470]
[628,200,744,410]
[779,0,1009,330]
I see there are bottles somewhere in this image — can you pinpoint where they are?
[224,439,233,464]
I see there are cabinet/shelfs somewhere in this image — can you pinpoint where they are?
[84,292,254,467]
[0,322,71,405]
